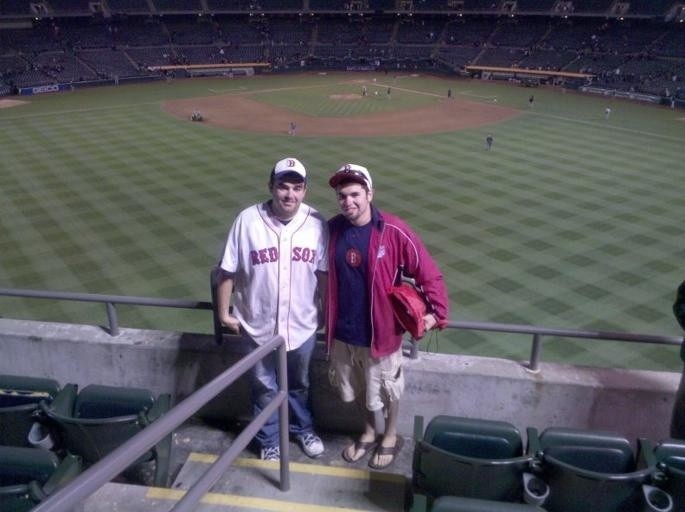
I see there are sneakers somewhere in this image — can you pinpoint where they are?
[260,446,280,460]
[296,431,325,457]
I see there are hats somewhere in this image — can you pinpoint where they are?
[270,157,307,183]
[329,163,372,190]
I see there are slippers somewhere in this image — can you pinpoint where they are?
[369,434,404,469]
[343,428,384,463]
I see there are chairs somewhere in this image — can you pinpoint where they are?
[413,415,534,500]
[41,383,171,486]
[0,447,82,512]
[534,426,661,512]
[0,375,61,449]
[0,0,684,99]
[409,495,534,512]
[645,438,685,511]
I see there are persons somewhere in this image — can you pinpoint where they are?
[0,16,685,149]
[670,280,685,443]
[217,157,331,464]
[312,162,448,469]
